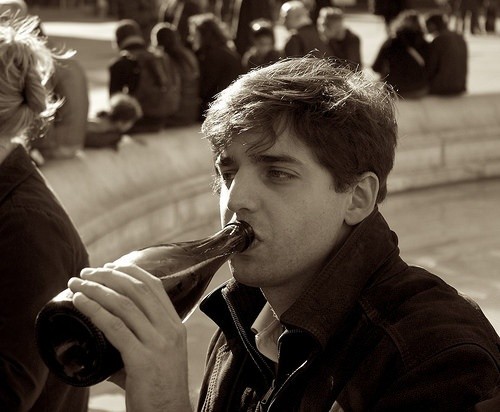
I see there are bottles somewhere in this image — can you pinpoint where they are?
[35,221,255,387]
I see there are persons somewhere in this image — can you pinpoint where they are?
[0,8,91,412]
[371,12,468,98]
[69,58,500,412]
[84,0,361,149]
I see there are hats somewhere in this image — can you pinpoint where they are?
[116,19,145,49]
